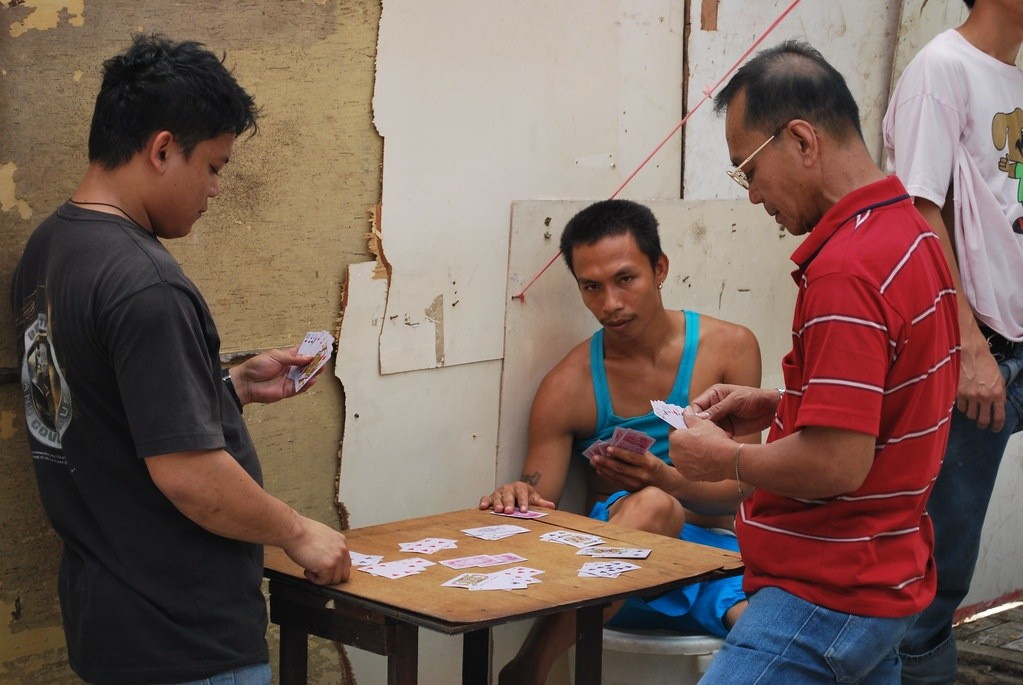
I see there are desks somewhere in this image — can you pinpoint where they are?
[261,503,746,685]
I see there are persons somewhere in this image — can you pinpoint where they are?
[669,40,960,685]
[11,31,352,685]
[881,0,1023,685]
[480,199,762,685]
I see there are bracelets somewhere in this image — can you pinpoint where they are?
[775,386,785,397]
[735,445,757,502]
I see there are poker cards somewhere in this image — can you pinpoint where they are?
[582,426,656,463]
[344,522,653,598]
[287,329,335,393]
[490,503,549,520]
[648,396,688,430]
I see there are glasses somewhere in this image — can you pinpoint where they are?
[726,116,797,190]
